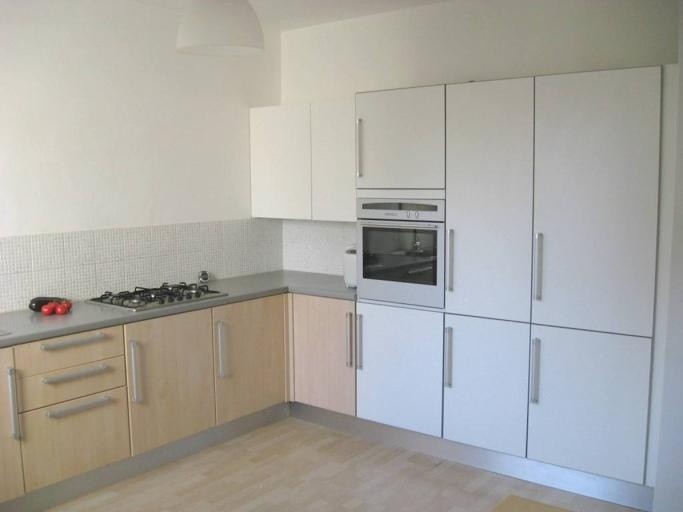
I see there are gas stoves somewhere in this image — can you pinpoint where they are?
[89,281,226,311]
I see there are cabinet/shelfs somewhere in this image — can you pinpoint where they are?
[444,76,536,325]
[291,292,354,417]
[442,312,531,461]
[526,324,653,480]
[530,65,662,339]
[248,100,356,223]
[123,293,286,456]
[3,324,132,503]
[358,83,446,193]
[356,301,445,440]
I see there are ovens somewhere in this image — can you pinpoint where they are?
[356,189,445,309]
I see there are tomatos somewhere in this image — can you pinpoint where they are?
[41,301,72,315]
